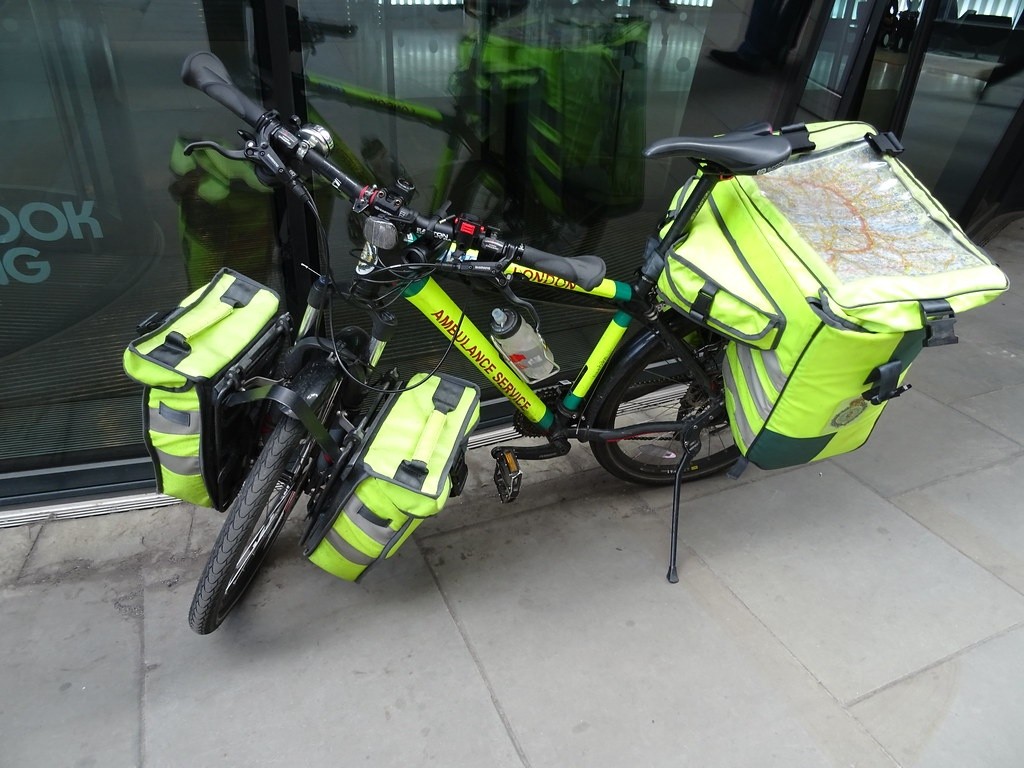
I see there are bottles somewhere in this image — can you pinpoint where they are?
[489,307,554,381]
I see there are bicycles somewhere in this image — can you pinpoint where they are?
[282,13,606,301]
[183,50,794,639]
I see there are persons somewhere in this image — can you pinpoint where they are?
[877,0,898,49]
[704,0,816,75]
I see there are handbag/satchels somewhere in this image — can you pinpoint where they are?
[297,374,479,582]
[122,266,294,511]
[170,137,333,291]
